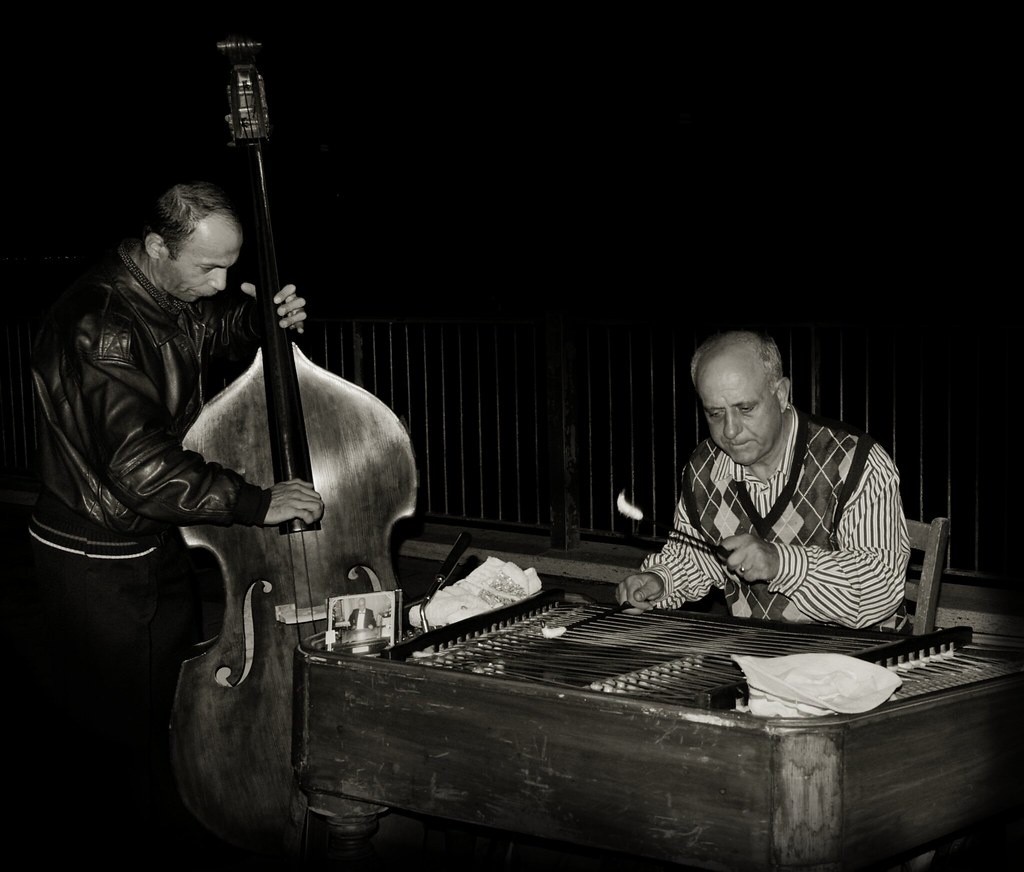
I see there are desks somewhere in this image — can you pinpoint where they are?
[294,587,1024,872]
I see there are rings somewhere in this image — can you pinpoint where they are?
[740,563,745,573]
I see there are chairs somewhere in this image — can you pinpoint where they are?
[896,516,948,636]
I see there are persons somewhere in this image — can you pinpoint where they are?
[615,334,910,629]
[0,181,325,872]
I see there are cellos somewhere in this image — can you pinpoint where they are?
[167,41,430,864]
